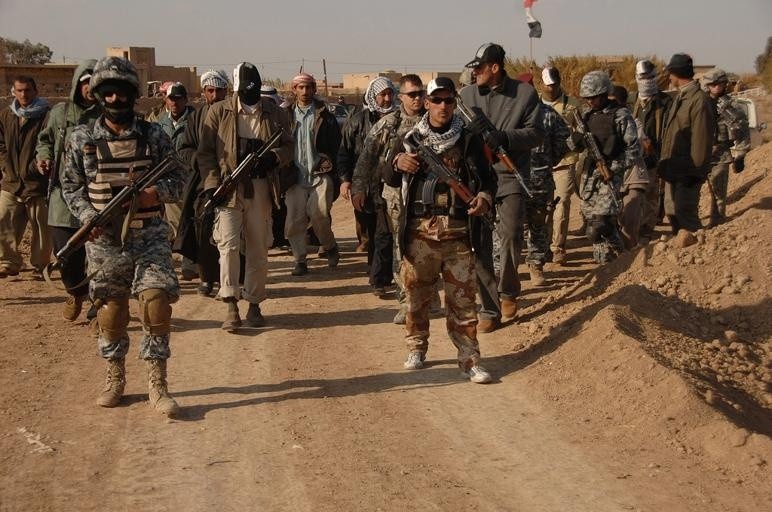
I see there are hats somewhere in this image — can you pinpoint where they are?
[159,81,174,92]
[165,83,190,99]
[79,69,94,83]
[541,67,562,86]
[609,86,628,103]
[465,40,506,68]
[666,53,695,78]
[200,69,230,89]
[233,62,262,94]
[291,73,318,92]
[426,76,457,96]
[337,95,345,100]
[260,85,284,104]
[636,59,658,78]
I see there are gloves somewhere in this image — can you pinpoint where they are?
[133,189,160,209]
[731,159,746,175]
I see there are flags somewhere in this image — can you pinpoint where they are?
[524,0,542,38]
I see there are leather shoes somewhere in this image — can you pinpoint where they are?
[476,318,496,335]
[373,286,386,300]
[198,280,214,294]
[502,299,518,321]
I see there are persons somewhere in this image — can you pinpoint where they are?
[493,73,571,297]
[259,85,290,250]
[455,43,543,333]
[61,57,188,413]
[198,63,295,330]
[351,74,425,297]
[338,77,397,253]
[538,67,581,265]
[703,70,750,228]
[335,96,348,115]
[284,73,342,276]
[177,69,246,294]
[0,75,54,278]
[626,60,674,240]
[608,85,650,248]
[146,82,179,122]
[567,71,640,265]
[153,84,198,245]
[383,76,495,384]
[659,52,714,237]
[457,68,477,93]
[35,59,100,323]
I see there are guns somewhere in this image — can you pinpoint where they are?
[571,107,620,209]
[634,117,659,166]
[44,161,55,202]
[50,150,177,273]
[404,130,496,231]
[456,92,534,199]
[199,121,288,220]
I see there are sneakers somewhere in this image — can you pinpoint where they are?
[461,365,492,384]
[290,263,307,276]
[278,242,289,249]
[326,249,339,268]
[30,267,46,280]
[553,246,567,264]
[245,310,266,327]
[527,264,546,286]
[394,308,406,325]
[317,246,327,258]
[222,315,243,333]
[403,350,426,372]
[62,293,83,323]
[0,268,19,280]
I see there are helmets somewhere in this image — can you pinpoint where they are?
[579,70,614,99]
[87,54,141,97]
[702,68,728,84]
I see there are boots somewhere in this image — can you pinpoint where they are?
[96,357,127,408]
[148,359,180,419]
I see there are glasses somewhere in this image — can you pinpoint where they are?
[102,89,129,100]
[425,95,458,106]
[402,90,426,98]
